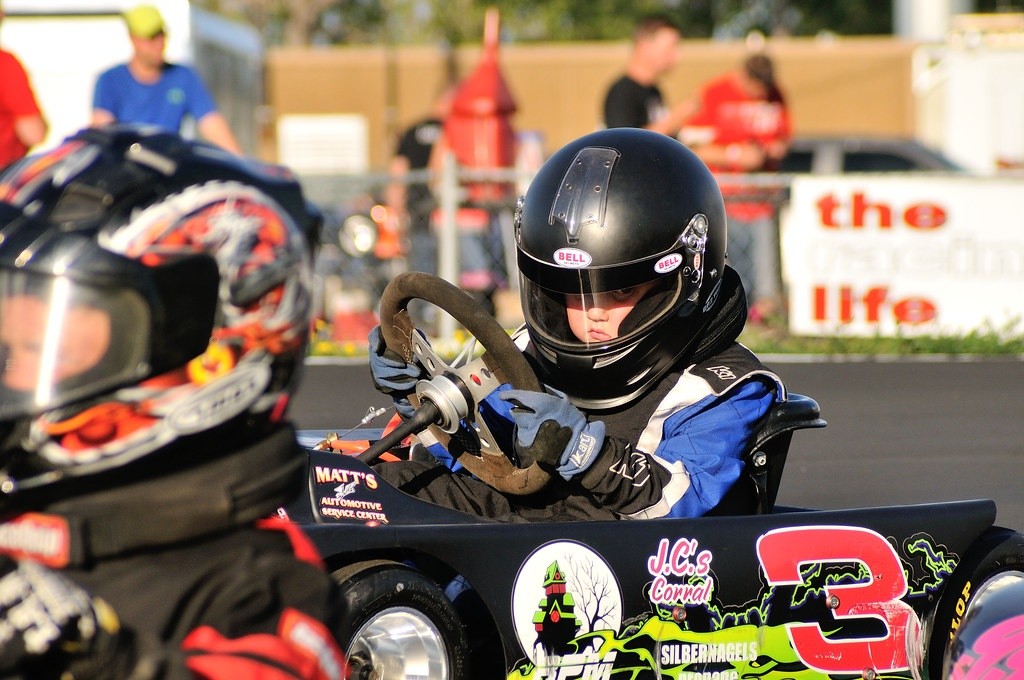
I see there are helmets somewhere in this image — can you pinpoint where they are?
[514,129,728,410]
[0,126,326,524]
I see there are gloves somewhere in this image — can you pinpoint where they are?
[504,384,606,482]
[368,325,434,419]
[0,558,120,680]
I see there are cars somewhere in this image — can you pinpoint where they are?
[774,134,966,171]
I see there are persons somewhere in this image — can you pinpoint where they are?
[363,129,784,518]
[0,0,793,348]
[0,117,362,680]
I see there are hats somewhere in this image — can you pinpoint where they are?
[126,5,167,40]
